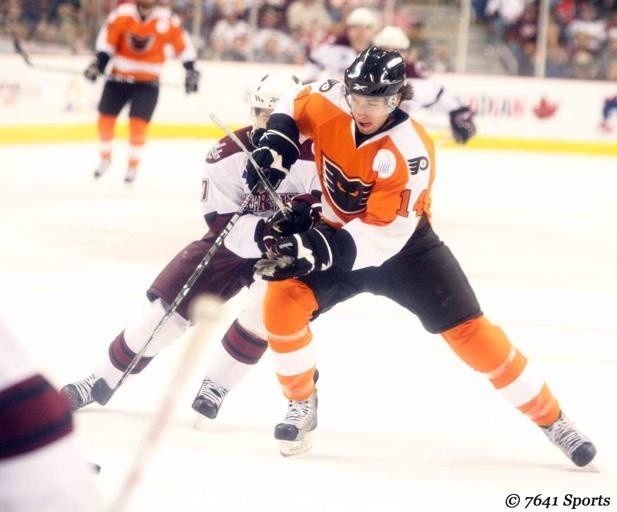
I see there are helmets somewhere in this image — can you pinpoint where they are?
[347,9,377,28]
[374,26,409,50]
[250,71,305,112]
[343,46,407,116]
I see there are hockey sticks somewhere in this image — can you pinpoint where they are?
[90,180,262,405]
[15,39,188,86]
[208,112,289,220]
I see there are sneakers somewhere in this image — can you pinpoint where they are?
[273,389,319,440]
[60,372,98,411]
[538,411,596,468]
[193,375,231,419]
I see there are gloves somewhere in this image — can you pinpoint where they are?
[254,202,334,282]
[85,62,103,80]
[185,71,198,93]
[240,114,301,195]
[449,106,475,144]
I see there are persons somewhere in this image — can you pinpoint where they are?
[1,1,616,143]
[53,70,322,420]
[78,2,201,186]
[239,41,599,472]
